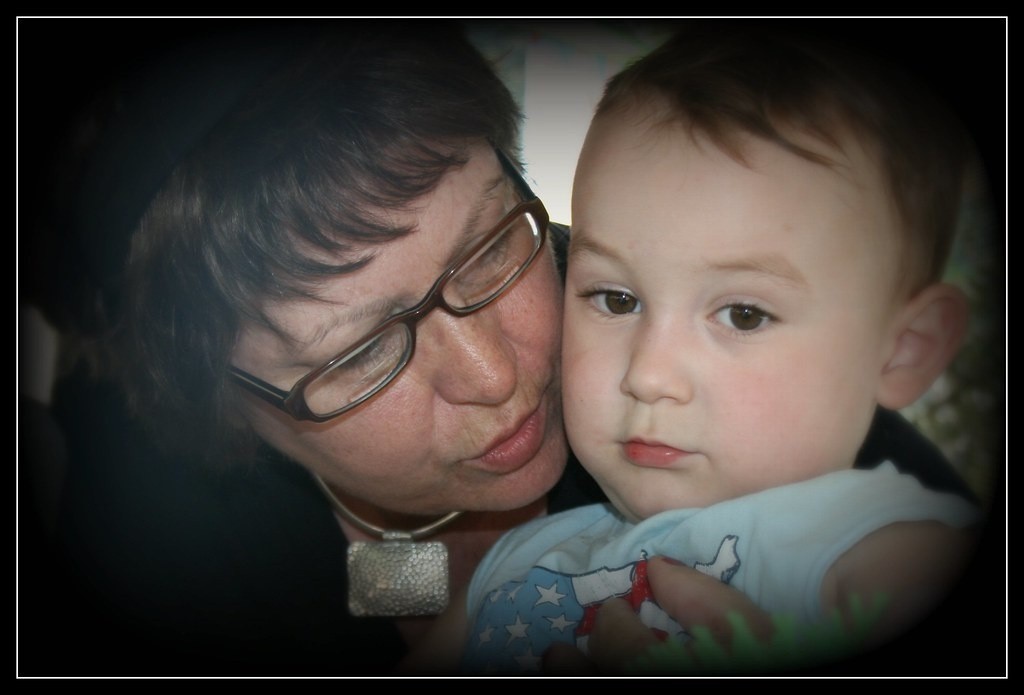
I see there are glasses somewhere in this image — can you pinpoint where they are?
[227,138,549,422]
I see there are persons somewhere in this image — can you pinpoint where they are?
[18,17,1007,677]
[399,16,988,678]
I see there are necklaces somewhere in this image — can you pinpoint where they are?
[310,473,468,618]
